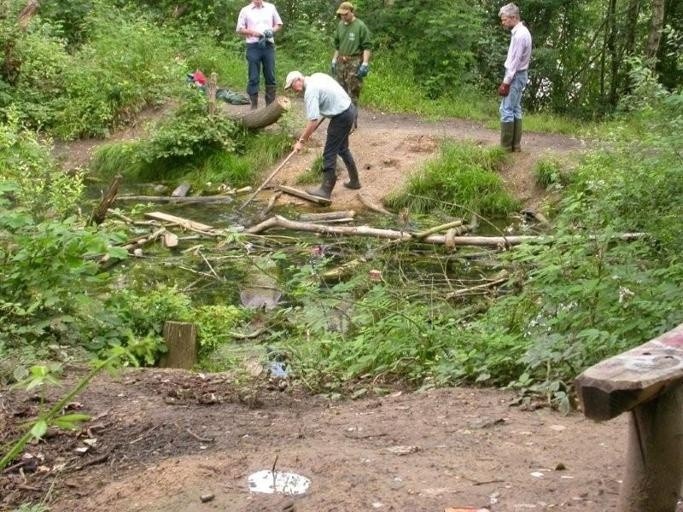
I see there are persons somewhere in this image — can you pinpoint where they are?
[236,0,284,113]
[330,2,373,129]
[496,3,533,153]
[284,71,362,200]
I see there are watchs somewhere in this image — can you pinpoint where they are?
[362,62,369,67]
[298,138,305,143]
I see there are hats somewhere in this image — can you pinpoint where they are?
[284,71,301,92]
[335,2,353,15]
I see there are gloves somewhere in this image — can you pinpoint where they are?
[258,35,265,50]
[263,29,272,39]
[331,61,336,78]
[356,64,368,80]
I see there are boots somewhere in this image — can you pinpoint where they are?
[343,166,360,189]
[305,171,336,199]
[512,117,522,152]
[348,102,358,134]
[499,121,514,154]
